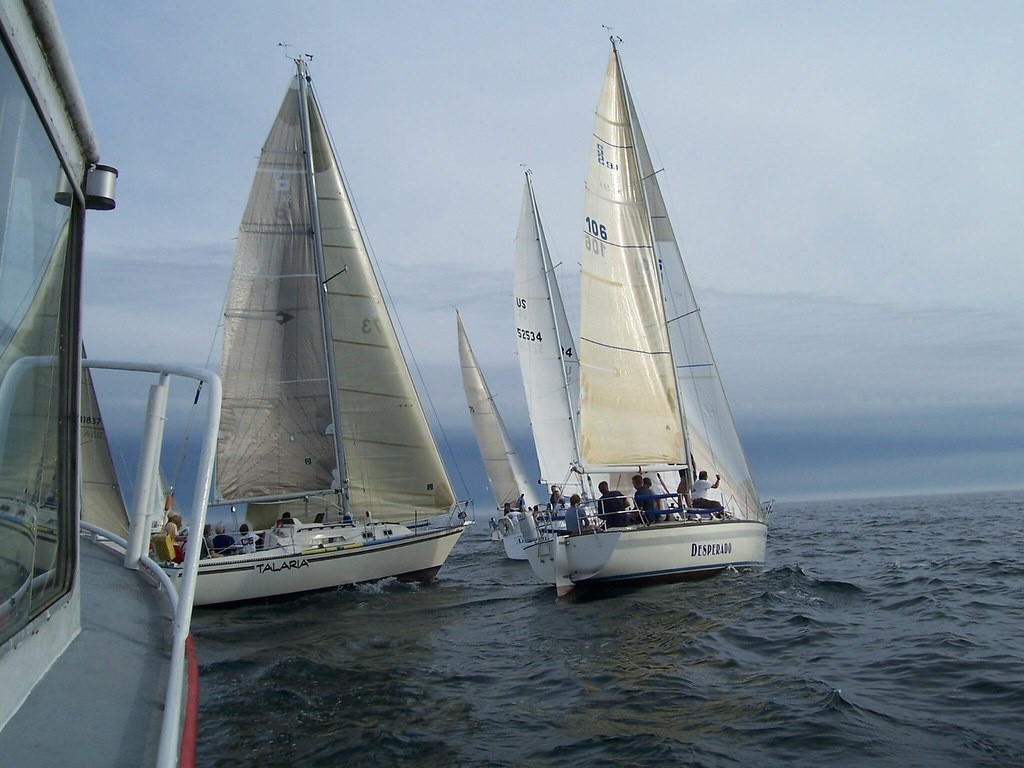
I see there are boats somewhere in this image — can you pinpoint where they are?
[1,0,222,768]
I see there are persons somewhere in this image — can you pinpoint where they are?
[565,494,601,535]
[163,511,297,565]
[504,485,565,532]
[598,452,730,530]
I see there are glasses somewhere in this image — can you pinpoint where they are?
[173,515,177,517]
[210,529,213,531]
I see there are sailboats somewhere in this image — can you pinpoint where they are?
[455,164,610,560]
[149,42,476,605]
[523,24,768,587]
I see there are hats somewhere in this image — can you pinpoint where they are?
[215,521,225,534]
[168,511,180,519]
